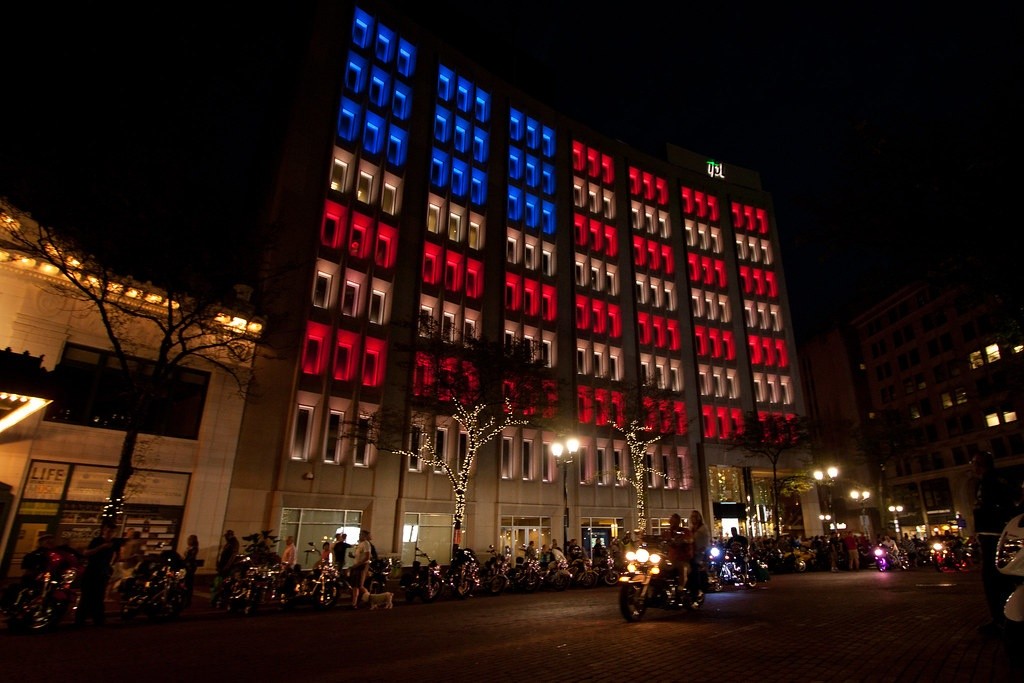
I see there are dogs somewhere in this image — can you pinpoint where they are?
[362,592,393,609]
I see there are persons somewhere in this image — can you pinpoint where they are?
[347,530,379,608]
[75,521,134,626]
[969,450,1017,638]
[594,538,602,548]
[749,535,775,551]
[934,530,955,542]
[660,509,710,603]
[211,529,241,609]
[280,535,297,570]
[525,541,536,560]
[110,528,145,561]
[777,535,815,553]
[541,538,568,569]
[564,540,582,561]
[812,531,871,573]
[319,531,354,575]
[902,533,923,568]
[882,535,901,567]
[727,526,749,583]
[184,534,199,608]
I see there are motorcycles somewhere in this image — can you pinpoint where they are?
[210,534,980,619]
[874,542,910,573]
[617,535,705,622]
[116,556,192,625]
[10,551,87,636]
